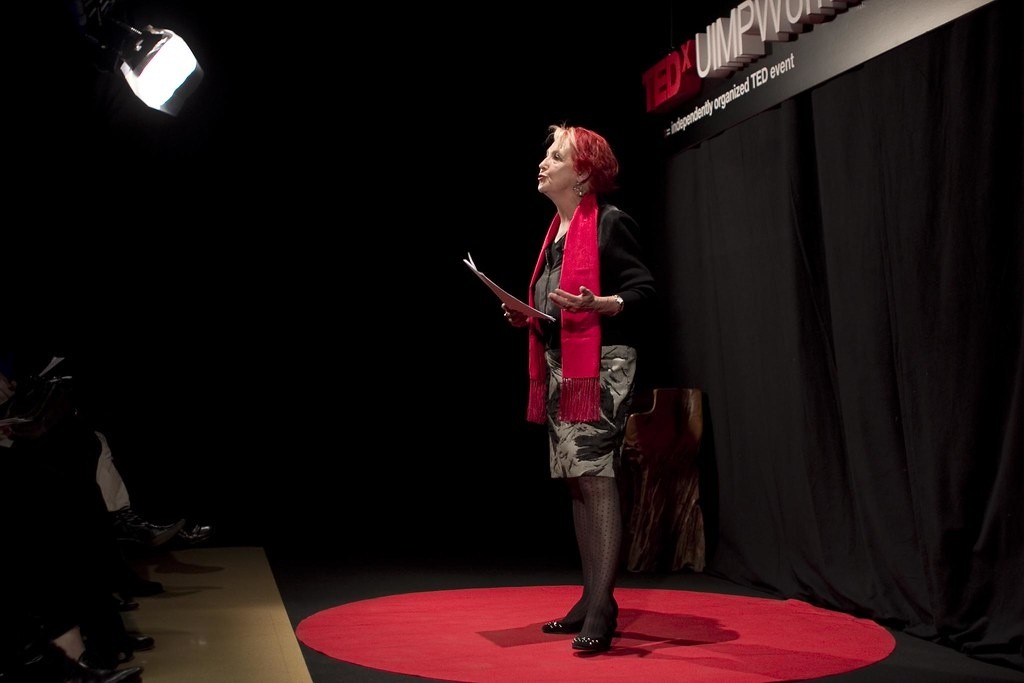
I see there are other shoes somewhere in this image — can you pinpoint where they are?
[115,598,139,613]
[123,633,154,650]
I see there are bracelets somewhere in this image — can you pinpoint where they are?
[609,295,623,317]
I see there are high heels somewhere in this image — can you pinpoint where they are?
[542,617,586,633]
[571,601,618,653]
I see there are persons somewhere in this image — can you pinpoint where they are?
[0,373,215,683]
[502,125,655,650]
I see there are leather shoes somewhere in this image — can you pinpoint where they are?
[121,570,163,598]
[170,523,211,548]
[66,650,143,683]
[108,508,184,546]
